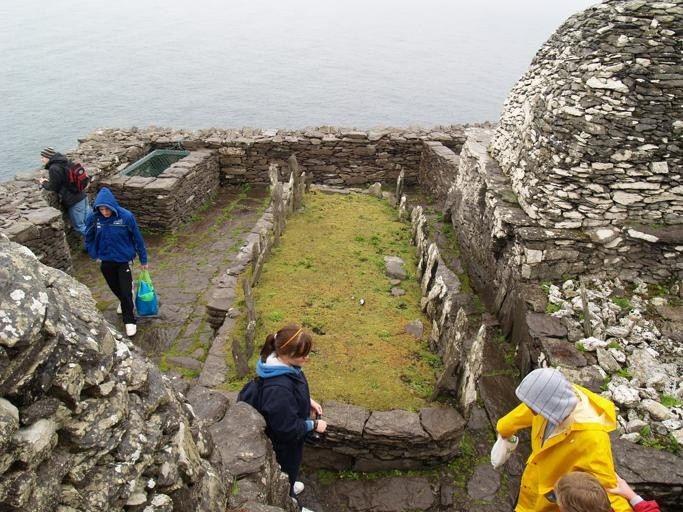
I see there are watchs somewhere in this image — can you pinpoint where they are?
[312,419,318,431]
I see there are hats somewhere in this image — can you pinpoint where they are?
[40,147,56,158]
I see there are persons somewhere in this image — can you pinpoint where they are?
[255,323,327,512]
[84,187,148,336]
[38,147,94,254]
[495,367,633,512]
[553,471,660,512]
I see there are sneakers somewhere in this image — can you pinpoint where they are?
[302,507,311,512]
[292,481,304,495]
[125,323,137,336]
[117,302,122,314]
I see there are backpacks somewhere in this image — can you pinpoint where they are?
[236,376,295,414]
[53,158,90,195]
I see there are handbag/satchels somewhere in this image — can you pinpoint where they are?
[135,269,159,316]
